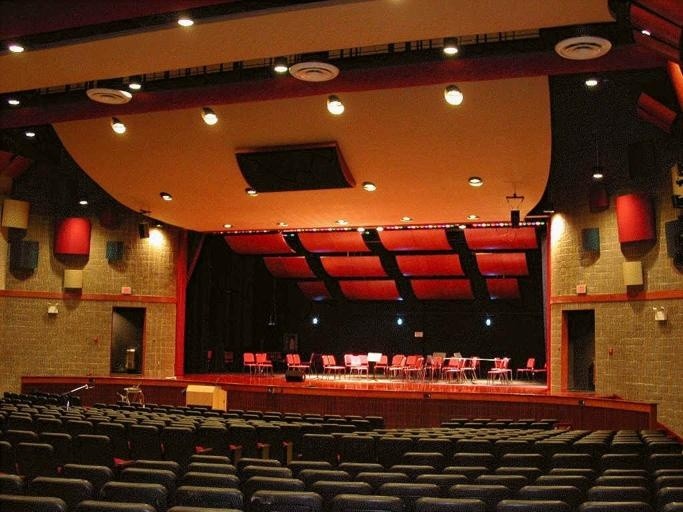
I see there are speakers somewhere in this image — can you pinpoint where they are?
[286,370,303,381]
[581,228,600,251]
[665,220,682,256]
[14,239,38,273]
[106,240,125,261]
